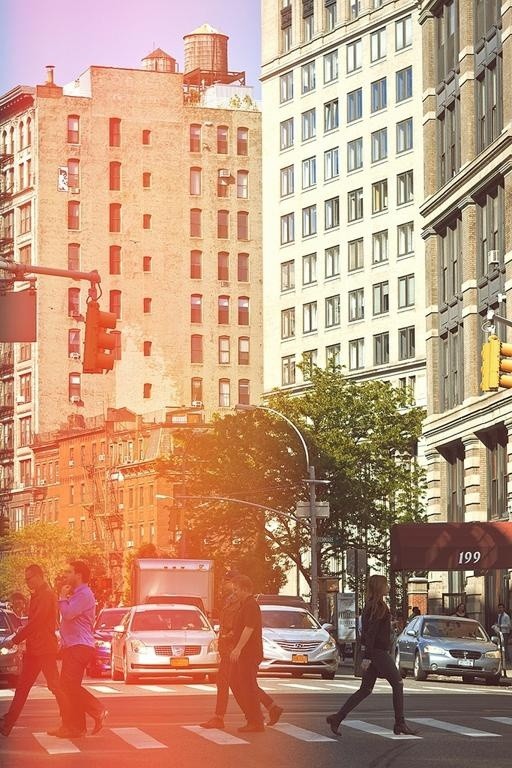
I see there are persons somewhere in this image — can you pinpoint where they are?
[410,607,421,618]
[46,560,108,737]
[451,603,468,617]
[199,580,283,728]
[327,575,415,735]
[358,607,362,641]
[495,603,510,660]
[1,565,61,737]
[228,574,263,733]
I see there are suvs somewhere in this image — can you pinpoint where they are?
[254,593,314,617]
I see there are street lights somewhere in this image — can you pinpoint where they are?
[156,495,186,559]
[235,404,318,619]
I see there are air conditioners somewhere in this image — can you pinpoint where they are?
[219,168,231,179]
[71,396,81,403]
[70,310,81,317]
[69,352,80,359]
[487,250,499,264]
[194,400,203,409]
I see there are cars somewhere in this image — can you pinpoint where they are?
[256,604,340,680]
[391,615,500,685]
[16,606,128,678]
[110,603,220,684]
[1,601,27,688]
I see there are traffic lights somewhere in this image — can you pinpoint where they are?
[488,339,511,389]
[83,301,117,374]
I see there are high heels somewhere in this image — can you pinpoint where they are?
[326,713,343,737]
[393,722,420,735]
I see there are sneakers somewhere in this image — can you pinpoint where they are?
[0,707,110,740]
[237,717,265,733]
[200,716,225,730]
[266,705,283,726]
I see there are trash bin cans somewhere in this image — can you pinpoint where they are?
[352,642,361,677]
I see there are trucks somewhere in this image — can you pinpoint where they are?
[336,591,364,658]
[130,558,219,634]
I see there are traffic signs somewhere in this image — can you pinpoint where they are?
[317,535,334,542]
